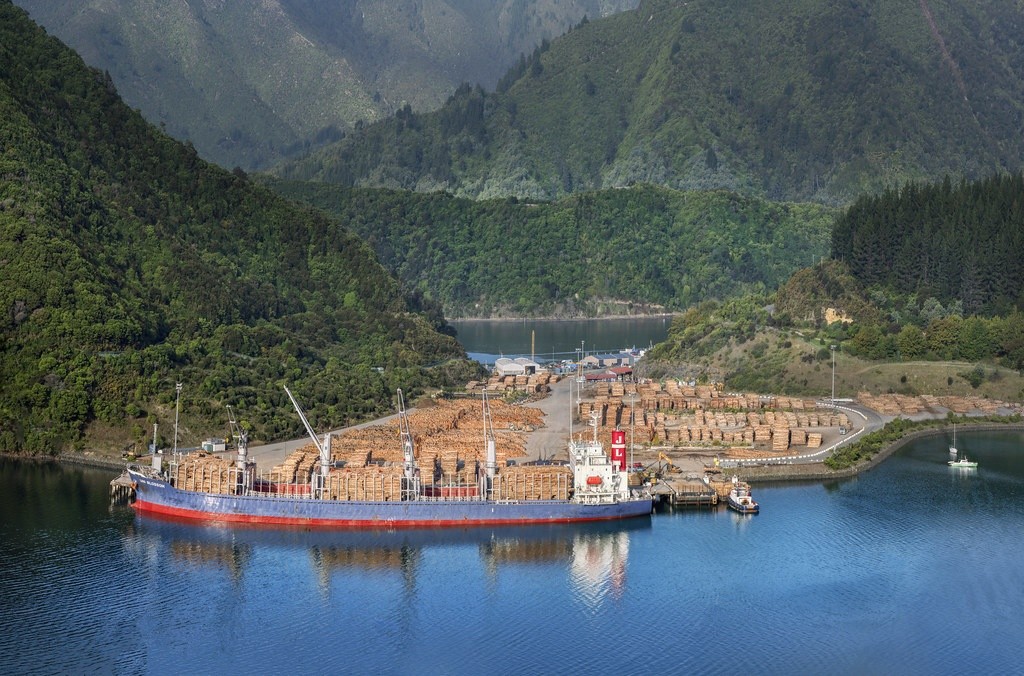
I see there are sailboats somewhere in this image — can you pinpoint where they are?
[948,423,958,461]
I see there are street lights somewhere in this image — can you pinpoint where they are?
[552,346,555,364]
[593,344,596,356]
[581,341,585,389]
[830,344,837,405]
[575,348,582,414]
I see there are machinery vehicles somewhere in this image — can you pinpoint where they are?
[704,454,722,474]
[655,451,683,479]
[628,454,659,472]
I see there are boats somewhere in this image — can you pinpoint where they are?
[948,456,979,467]
[125,381,654,527]
[727,486,759,515]
